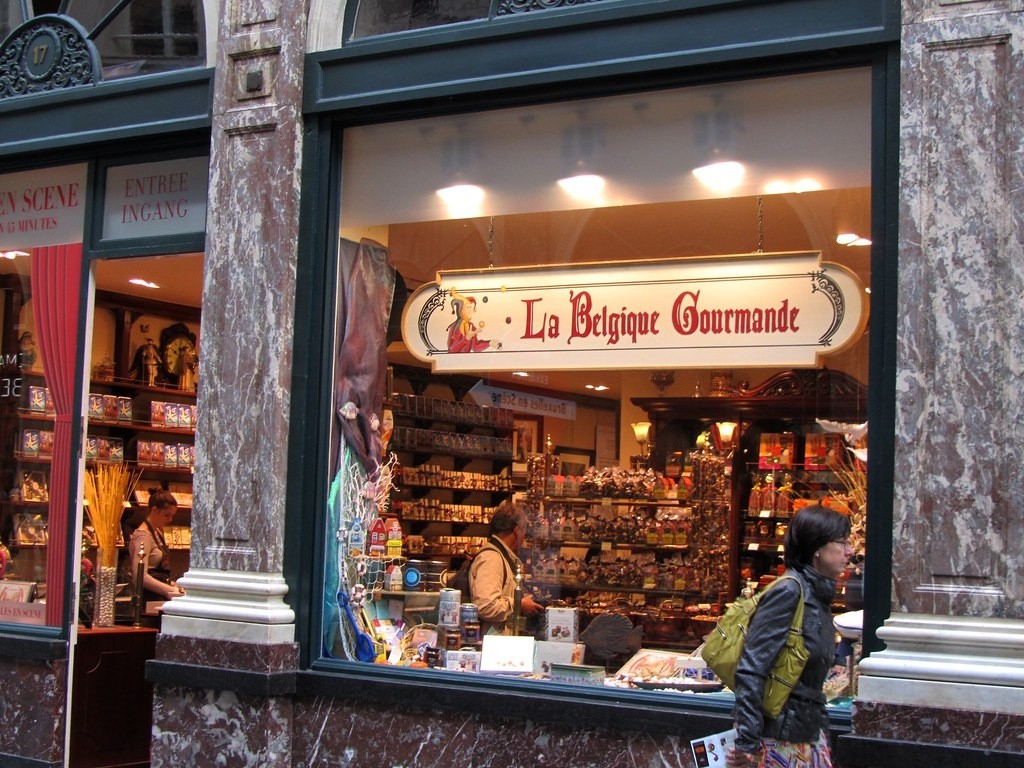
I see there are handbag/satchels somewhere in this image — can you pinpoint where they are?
[699,572,810,718]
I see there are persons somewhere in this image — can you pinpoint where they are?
[722,504,855,766]
[128,486,185,610]
[466,503,544,651]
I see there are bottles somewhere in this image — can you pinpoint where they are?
[748,472,793,517]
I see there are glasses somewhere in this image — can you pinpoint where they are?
[827,536,854,549]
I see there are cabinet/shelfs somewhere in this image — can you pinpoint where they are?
[1,363,867,642]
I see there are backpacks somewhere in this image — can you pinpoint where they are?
[443,548,510,608]
